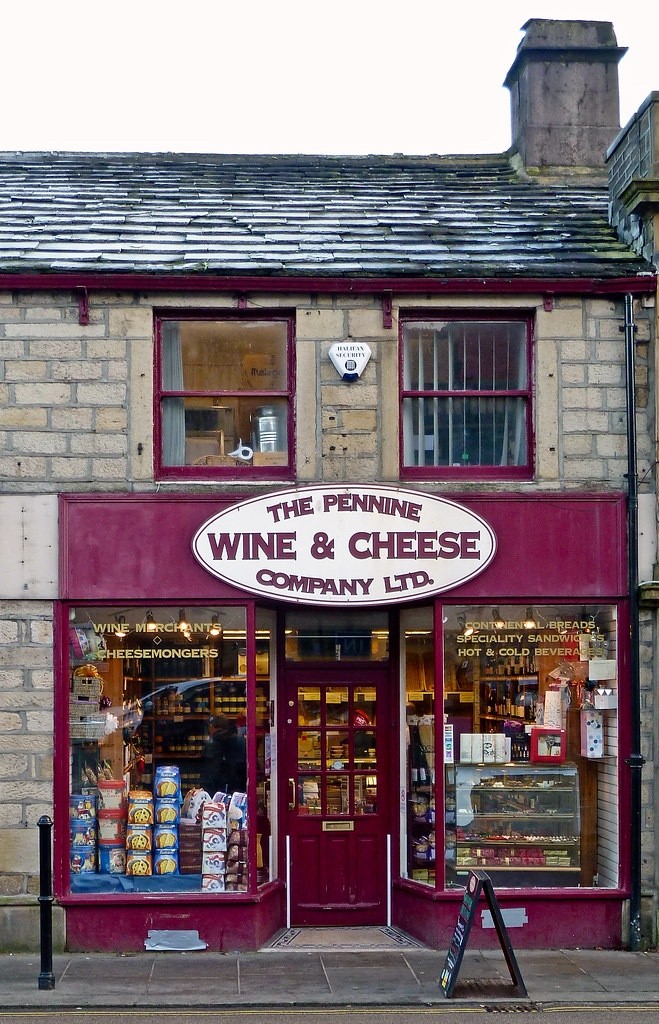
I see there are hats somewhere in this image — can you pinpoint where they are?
[209,712,227,728]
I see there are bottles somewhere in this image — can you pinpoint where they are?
[511,743,529,760]
[486,653,534,676]
[486,684,524,718]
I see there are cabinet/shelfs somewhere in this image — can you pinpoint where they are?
[95,626,272,818]
[446,629,598,887]
[298,641,481,765]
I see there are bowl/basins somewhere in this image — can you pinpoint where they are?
[332,746,343,749]
[330,750,344,753]
[330,754,342,758]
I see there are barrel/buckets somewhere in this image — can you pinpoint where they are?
[69,795,96,873]
[98,813,126,844]
[98,845,126,874]
[95,780,125,812]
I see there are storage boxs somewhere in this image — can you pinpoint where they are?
[529,729,564,764]
[581,709,605,758]
[299,781,342,815]
[69,676,107,740]
[237,647,268,675]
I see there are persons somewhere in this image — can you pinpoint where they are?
[198,712,246,799]
